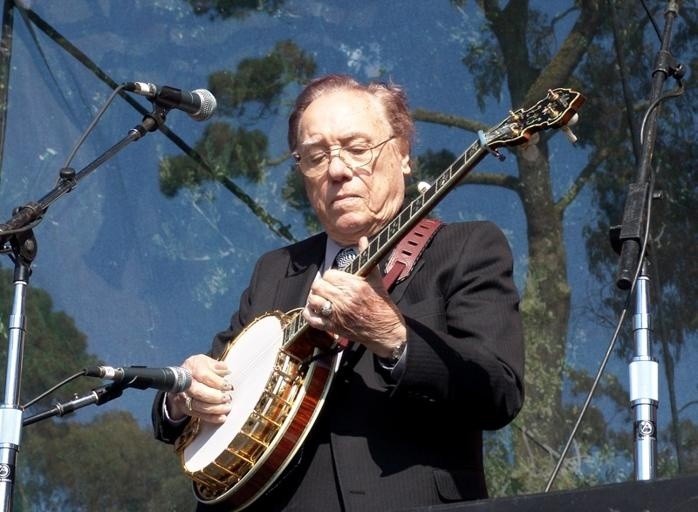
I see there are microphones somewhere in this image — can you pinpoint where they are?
[146,83,218,121]
[101,365,192,395]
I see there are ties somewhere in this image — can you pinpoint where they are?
[334,248,358,274]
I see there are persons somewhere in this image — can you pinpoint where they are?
[150,72,525,512]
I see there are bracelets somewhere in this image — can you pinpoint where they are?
[389,339,408,366]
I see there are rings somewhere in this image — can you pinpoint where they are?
[321,301,332,315]
[184,396,193,412]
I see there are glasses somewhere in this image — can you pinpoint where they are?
[295,133,401,180]
